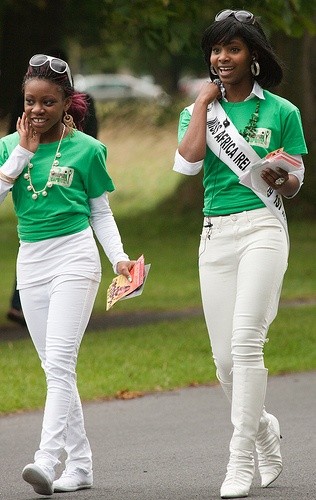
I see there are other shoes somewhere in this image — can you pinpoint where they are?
[6,311,28,327]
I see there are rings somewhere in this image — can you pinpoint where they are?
[275,177,285,184]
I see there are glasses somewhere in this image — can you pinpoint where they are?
[29,54,72,87]
[215,9,266,39]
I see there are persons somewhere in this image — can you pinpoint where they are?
[173,9,308,498]
[5,51,99,326]
[0,54,145,495]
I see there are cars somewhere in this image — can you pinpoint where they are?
[74,75,168,103]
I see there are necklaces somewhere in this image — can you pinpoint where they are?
[239,96,260,141]
[24,123,65,199]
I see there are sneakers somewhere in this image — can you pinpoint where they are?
[22,465,53,496]
[53,470,93,491]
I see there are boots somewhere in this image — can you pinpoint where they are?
[216,369,282,487]
[220,368,269,497]
